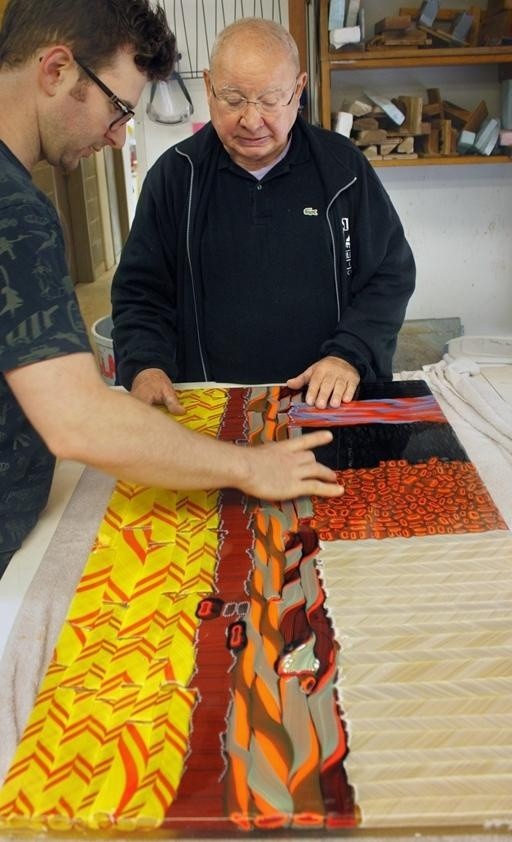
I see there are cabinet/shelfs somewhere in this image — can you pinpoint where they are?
[315,0,511,168]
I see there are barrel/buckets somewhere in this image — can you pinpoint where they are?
[91,314,116,387]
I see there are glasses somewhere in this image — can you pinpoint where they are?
[205,75,297,108]
[69,45,135,132]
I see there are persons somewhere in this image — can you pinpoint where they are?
[110,16,416,415]
[0,0,345,579]
